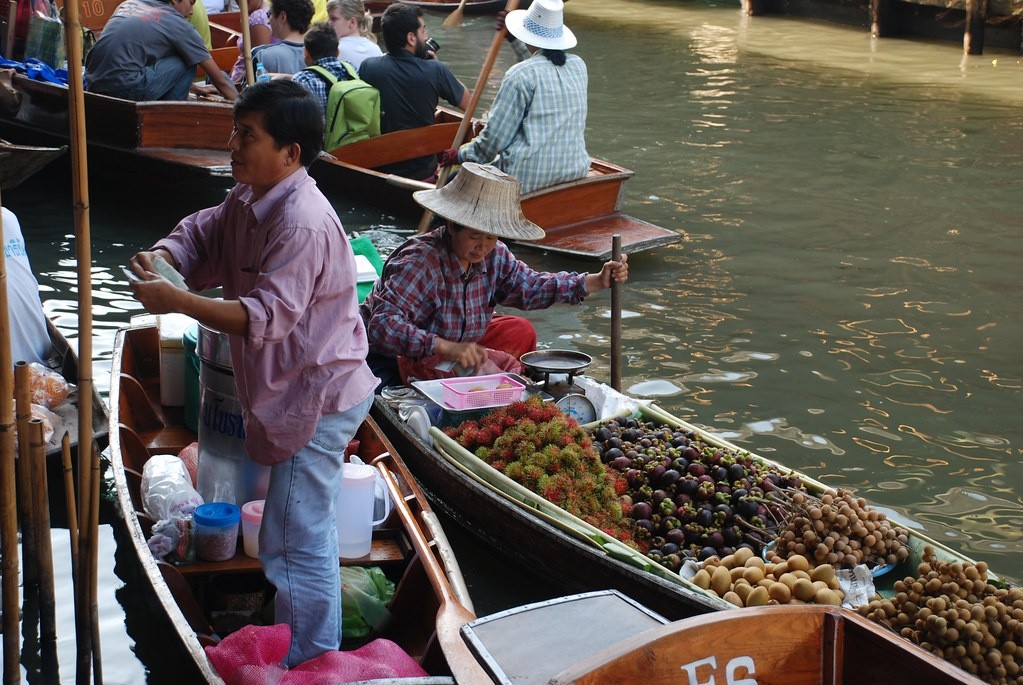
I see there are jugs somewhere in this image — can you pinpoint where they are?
[337,462,390,559]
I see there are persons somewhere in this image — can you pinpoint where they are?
[240,0,316,90]
[257,22,381,153]
[230,0,282,83]
[359,161,628,370]
[439,0,591,195]
[359,3,471,135]
[326,0,384,70]
[125,80,382,670]
[84,0,238,101]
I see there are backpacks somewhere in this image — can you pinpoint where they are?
[300,61,381,153]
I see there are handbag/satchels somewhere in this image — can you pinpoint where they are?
[23,0,62,70]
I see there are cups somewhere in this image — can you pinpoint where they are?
[172,516,198,566]
[240,500,267,558]
[193,501,241,561]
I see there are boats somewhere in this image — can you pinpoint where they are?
[107,324,480,685]
[1,11,247,177]
[14,316,110,488]
[313,105,685,262]
[460,588,987,685]
[360,0,570,18]
[372,364,1022,685]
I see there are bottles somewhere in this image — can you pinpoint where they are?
[255,63,270,84]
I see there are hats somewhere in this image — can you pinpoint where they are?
[412,162,545,240]
[505,0,577,50]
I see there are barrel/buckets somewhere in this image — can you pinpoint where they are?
[181,322,200,433]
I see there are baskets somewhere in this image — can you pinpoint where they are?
[439,375,525,409]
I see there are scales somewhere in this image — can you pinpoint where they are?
[519,349,598,426]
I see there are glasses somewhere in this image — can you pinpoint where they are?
[266,9,274,18]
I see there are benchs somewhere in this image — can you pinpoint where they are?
[166,527,414,571]
[323,119,616,232]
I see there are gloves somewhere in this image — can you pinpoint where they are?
[439,149,458,167]
[495,9,516,42]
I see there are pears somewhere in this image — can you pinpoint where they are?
[687,546,849,609]
